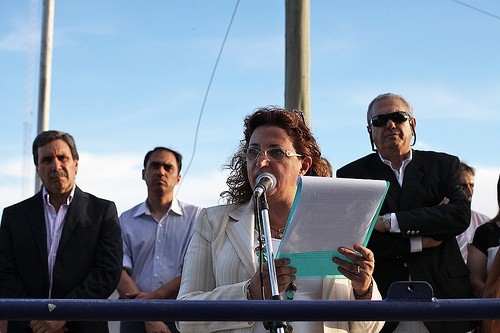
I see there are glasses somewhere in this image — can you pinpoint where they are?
[245,147,307,162]
[370,112,411,126]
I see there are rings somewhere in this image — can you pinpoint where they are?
[354,265,360,275]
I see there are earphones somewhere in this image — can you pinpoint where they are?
[409,120,413,125]
[368,128,372,132]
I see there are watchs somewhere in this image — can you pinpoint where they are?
[383,213,390,231]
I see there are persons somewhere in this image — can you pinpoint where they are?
[0,131,124,333]
[177,108,387,333]
[116,147,202,333]
[455,162,493,265]
[336,92,472,333]
[467,174,500,333]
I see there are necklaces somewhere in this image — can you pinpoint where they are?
[270,226,284,239]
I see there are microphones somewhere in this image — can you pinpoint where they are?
[254,172,277,198]
